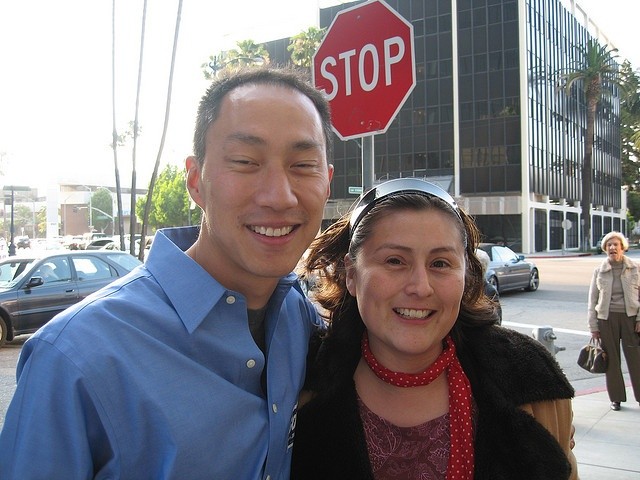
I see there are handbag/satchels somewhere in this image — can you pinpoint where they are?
[577,336,611,375]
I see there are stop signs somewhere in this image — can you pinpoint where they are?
[311,0,416,141]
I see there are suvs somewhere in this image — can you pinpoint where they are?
[13,236,31,249]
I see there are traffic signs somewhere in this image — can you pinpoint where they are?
[349,187,362,193]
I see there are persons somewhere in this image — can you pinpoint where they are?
[291,177,578,480]
[0,67,327,480]
[588,231,640,410]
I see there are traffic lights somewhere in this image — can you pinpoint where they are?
[72,205,77,214]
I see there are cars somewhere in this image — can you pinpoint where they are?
[0,250,144,348]
[83,239,114,249]
[474,243,540,295]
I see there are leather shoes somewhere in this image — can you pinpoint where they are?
[611,401,623,411]
